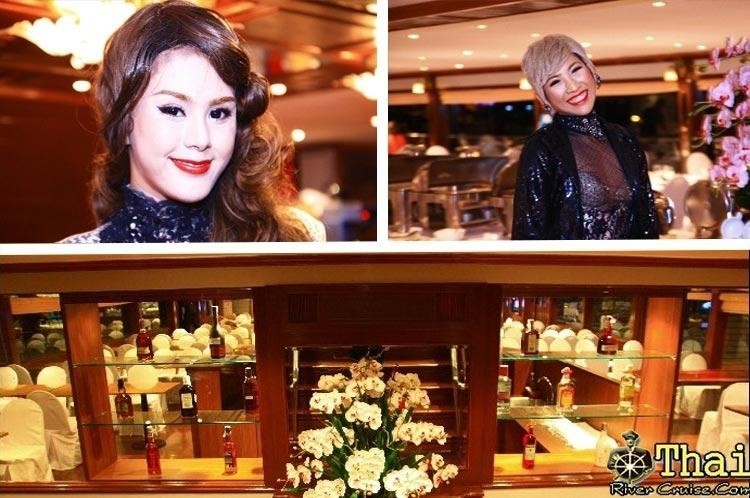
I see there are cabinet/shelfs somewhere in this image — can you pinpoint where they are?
[498,319,749,476]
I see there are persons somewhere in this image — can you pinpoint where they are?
[509,35,660,239]
[388,112,411,229]
[48,0,316,242]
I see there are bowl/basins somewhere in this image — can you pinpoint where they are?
[433,228,467,241]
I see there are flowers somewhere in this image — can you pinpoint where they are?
[685,37,749,209]
[276,352,460,498]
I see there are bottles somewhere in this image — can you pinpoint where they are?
[142,419,161,475]
[114,367,258,425]
[496,365,513,415]
[222,425,239,475]
[135,317,155,361]
[556,366,576,414]
[208,305,226,360]
[593,422,611,468]
[597,316,618,356]
[521,423,538,469]
[618,364,637,416]
[520,318,540,355]
